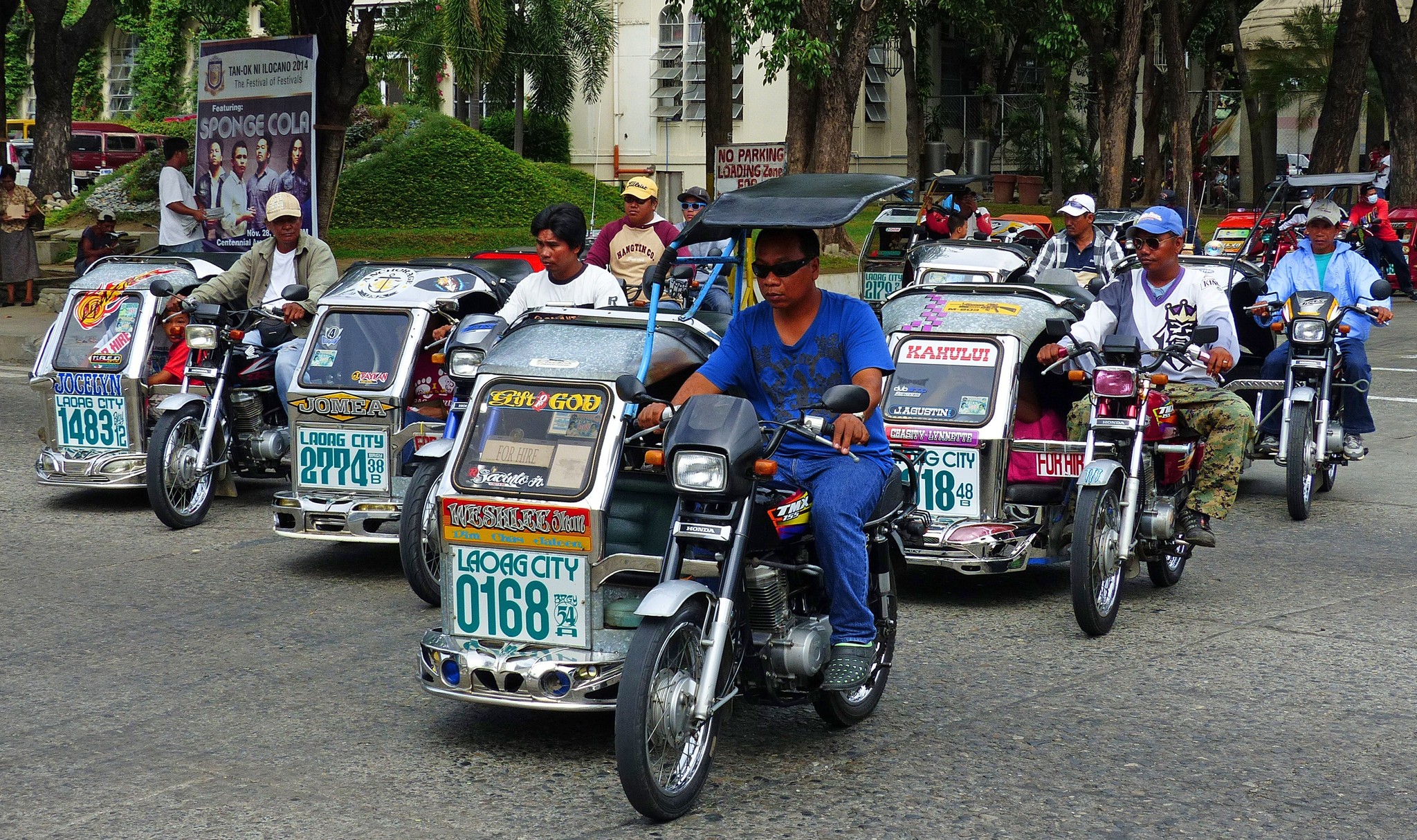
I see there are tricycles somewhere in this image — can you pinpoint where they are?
[1262,181,1367,283]
[901,236,1102,297]
[1125,171,1393,519]
[466,250,696,313]
[1084,209,1196,258]
[1204,211,1285,262]
[1370,205,1417,297]
[856,174,995,316]
[879,282,1225,638]
[984,212,1052,255]
[29,254,294,528]
[270,258,511,608]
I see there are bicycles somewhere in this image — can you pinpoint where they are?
[81,223,162,277]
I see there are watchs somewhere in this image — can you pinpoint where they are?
[975,210,979,214]
[852,412,865,423]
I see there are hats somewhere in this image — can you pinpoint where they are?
[1056,193,1096,216]
[1128,206,1183,236]
[1299,185,1314,198]
[675,186,711,205]
[620,176,658,200]
[1306,198,1341,226]
[97,210,116,221]
[263,192,302,221]
[1155,190,1175,205]
[955,187,976,204]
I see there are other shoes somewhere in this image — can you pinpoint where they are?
[816,632,877,690]
[21,299,35,306]
[281,444,292,465]
[2,302,15,307]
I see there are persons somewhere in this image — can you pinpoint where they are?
[1154,189,1205,256]
[1368,140,1391,199]
[673,186,735,315]
[1369,145,1387,182]
[1348,184,1417,302]
[246,132,279,242]
[196,139,227,251]
[1258,187,1316,236]
[1035,206,1256,547]
[159,137,205,253]
[920,366,1068,484]
[933,169,960,212]
[166,192,338,418]
[1252,200,1393,461]
[393,319,456,464]
[637,227,897,692]
[74,210,117,278]
[947,212,968,240]
[582,176,696,310]
[278,135,311,227]
[1025,193,1129,288]
[0,164,41,307]
[1131,162,1240,209]
[923,187,992,242]
[146,312,211,427]
[80,317,173,387]
[220,141,256,252]
[433,204,629,344]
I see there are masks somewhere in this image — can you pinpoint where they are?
[1361,194,1377,204]
[1300,197,1314,208]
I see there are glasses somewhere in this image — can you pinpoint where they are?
[678,202,707,210]
[1062,200,1091,212]
[1131,236,1179,251]
[623,197,651,204]
[1366,184,1375,189]
[751,256,811,279]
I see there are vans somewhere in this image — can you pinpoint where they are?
[1288,154,1312,175]
[69,121,171,182]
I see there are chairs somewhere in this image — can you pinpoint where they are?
[148,323,172,376]
[1005,381,1094,506]
[601,468,685,588]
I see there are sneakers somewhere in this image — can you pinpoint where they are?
[1259,434,1279,453]
[1179,506,1216,547]
[1406,291,1417,302]
[1342,432,1364,460]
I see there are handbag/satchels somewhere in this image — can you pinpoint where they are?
[258,316,296,347]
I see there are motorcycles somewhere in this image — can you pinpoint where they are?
[1122,165,1232,205]
[414,174,932,820]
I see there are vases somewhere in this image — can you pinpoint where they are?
[1015,175,1044,206]
[992,174,1016,204]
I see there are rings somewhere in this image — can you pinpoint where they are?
[1223,360,1228,364]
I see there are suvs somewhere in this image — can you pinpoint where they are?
[5,119,36,142]
[6,137,35,189]
